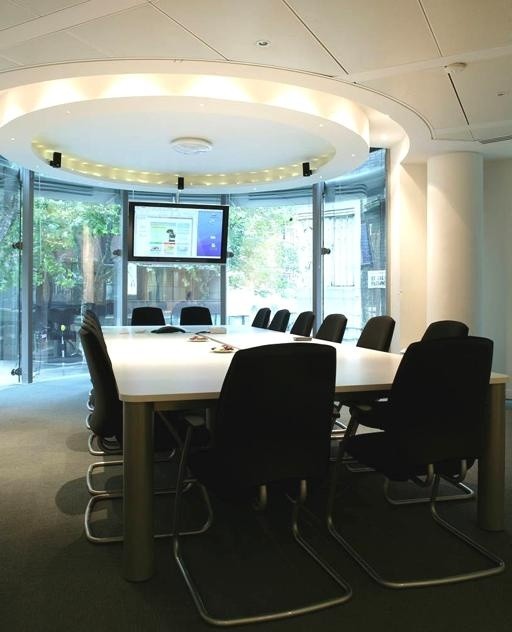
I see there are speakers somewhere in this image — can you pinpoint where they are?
[303,163,312,176]
[178,178,184,189]
[50,152,61,168]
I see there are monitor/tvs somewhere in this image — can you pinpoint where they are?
[128,202,229,263]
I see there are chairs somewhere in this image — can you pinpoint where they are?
[350,319,476,507]
[83,318,180,458]
[267,308,291,333]
[251,307,272,329]
[314,312,348,435]
[288,310,317,337]
[169,342,356,628]
[97,323,511,561]
[78,327,214,545]
[131,306,166,325]
[328,314,396,467]
[324,334,507,590]
[78,322,194,494]
[179,306,213,325]
[83,309,104,430]
